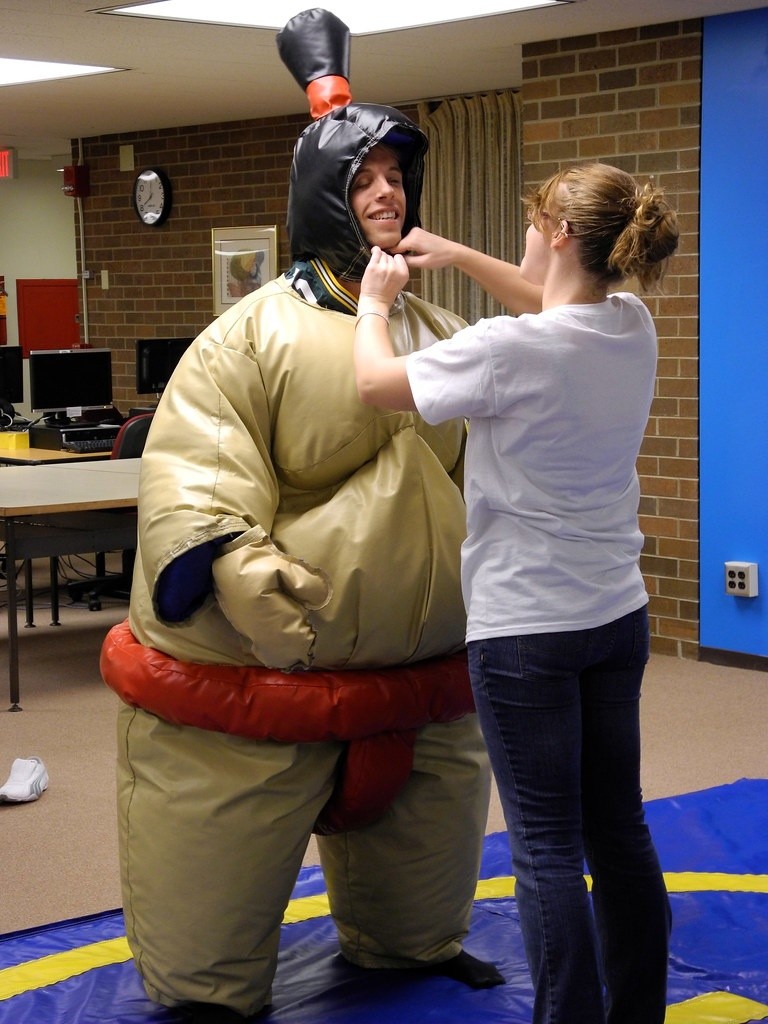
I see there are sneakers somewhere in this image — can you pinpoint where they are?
[1,756,45,804]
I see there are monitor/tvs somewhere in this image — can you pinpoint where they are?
[136,337,196,394]
[0,345,23,403]
[28,348,112,428]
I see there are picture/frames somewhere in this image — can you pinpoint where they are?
[211,225,279,316]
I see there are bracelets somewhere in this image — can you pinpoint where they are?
[354,313,392,328]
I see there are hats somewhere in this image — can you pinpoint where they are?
[275,8,428,286]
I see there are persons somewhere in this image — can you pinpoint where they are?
[357,159,679,1024]
[115,106,506,1022]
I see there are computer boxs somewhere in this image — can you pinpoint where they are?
[29,424,121,450]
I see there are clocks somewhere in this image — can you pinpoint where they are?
[133,168,171,226]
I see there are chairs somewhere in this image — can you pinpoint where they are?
[69,414,155,612]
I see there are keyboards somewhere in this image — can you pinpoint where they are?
[66,439,116,452]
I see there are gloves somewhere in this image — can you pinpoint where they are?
[209,524,325,673]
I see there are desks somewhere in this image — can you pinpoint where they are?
[0,458,140,712]
[0,447,112,628]
